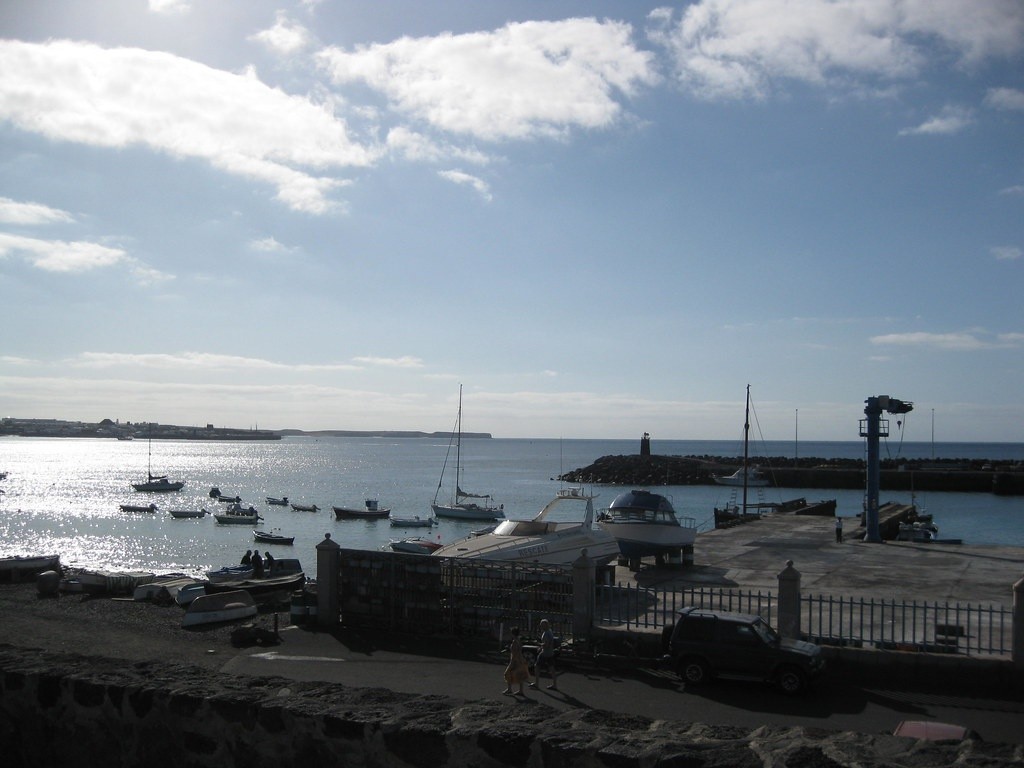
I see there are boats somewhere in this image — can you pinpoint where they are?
[117,384,506,555]
[430,495,621,589]
[711,466,771,486]
[556,473,601,499]
[0,555,306,628]
[595,490,698,558]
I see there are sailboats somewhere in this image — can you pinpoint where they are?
[714,384,837,529]
[899,469,938,539]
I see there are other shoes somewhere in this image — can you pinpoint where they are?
[514,690,523,694]
[528,682,538,688]
[502,688,512,694]
[547,684,557,689]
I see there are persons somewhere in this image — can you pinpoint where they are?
[529,619,558,689]
[241,550,252,564]
[265,552,281,577]
[835,517,842,543]
[252,550,264,579]
[503,627,524,695]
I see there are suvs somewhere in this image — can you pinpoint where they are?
[661,607,824,695]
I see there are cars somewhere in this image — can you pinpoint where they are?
[982,464,993,470]
[1009,461,1024,471]
